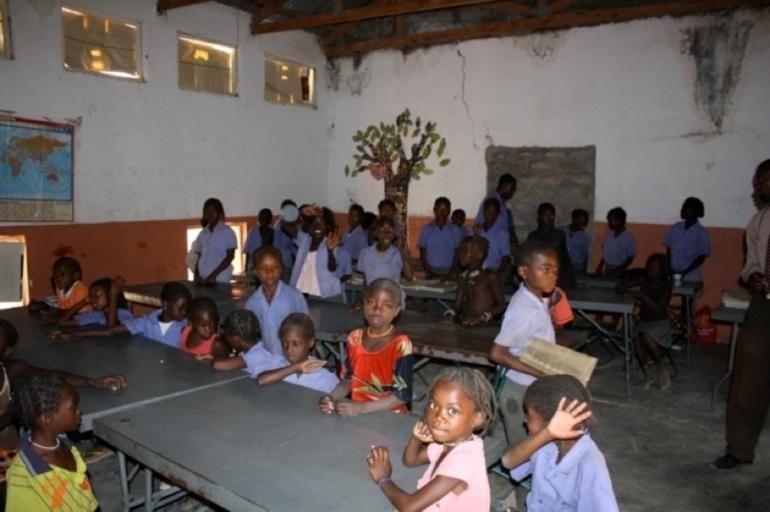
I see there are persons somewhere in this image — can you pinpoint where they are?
[0,238,622,510]
[662,198,710,322]
[615,253,673,390]
[714,159,770,470]
[192,173,638,327]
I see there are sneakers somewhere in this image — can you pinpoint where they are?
[715,454,753,470]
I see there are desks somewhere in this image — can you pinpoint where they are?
[2,306,258,511]
[334,314,587,428]
[347,257,744,401]
[91,370,524,510]
[113,281,372,385]
